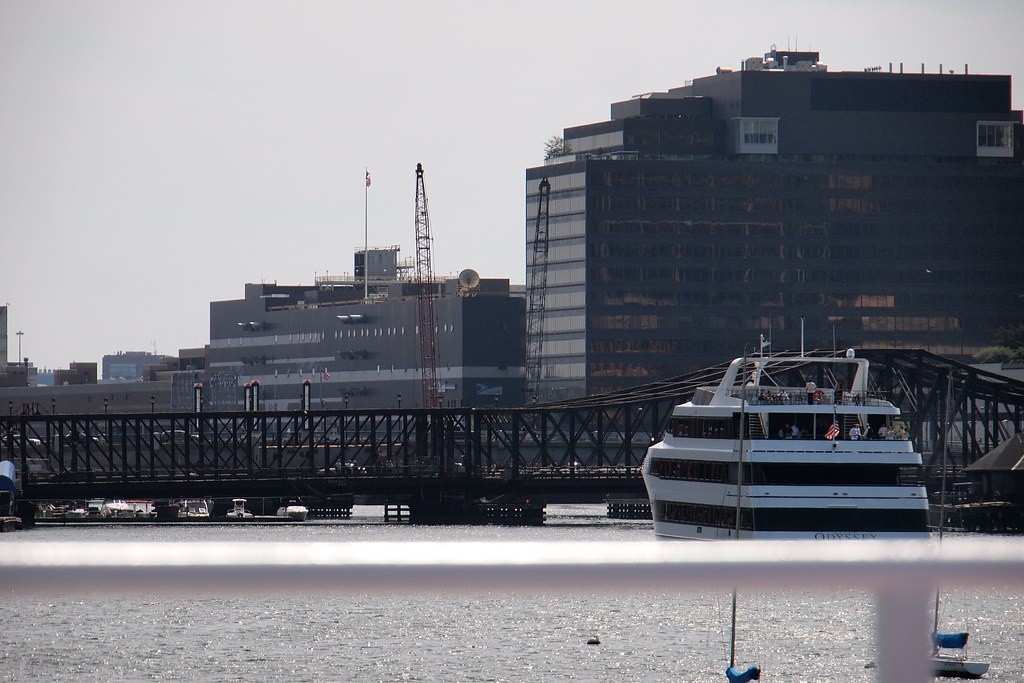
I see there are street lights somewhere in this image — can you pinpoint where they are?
[439,394,443,409]
[16,331,24,366]
[104,397,109,437]
[150,395,154,416]
[199,395,204,413]
[248,393,253,411]
[396,393,402,442]
[300,394,304,410]
[345,395,348,441]
[532,396,537,441]
[8,400,13,431]
[52,396,56,445]
[494,393,499,438]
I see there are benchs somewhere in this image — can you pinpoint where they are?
[729,390,879,406]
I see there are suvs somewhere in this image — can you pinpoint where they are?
[162,431,200,443]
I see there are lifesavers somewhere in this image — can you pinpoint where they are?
[813,390,825,403]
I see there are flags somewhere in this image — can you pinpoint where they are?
[825,410,839,440]
[367,172,371,187]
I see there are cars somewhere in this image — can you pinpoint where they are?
[316,464,369,476]
[2,431,101,446]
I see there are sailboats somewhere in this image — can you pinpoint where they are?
[928,587,989,677]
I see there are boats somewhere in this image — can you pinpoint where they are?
[640,311,936,541]
[1001,359,1024,370]
[31,495,309,522]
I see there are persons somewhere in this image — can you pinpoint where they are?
[833,379,843,404]
[758,389,789,405]
[848,424,909,440]
[778,422,810,439]
[806,379,817,405]
[336,459,358,474]
